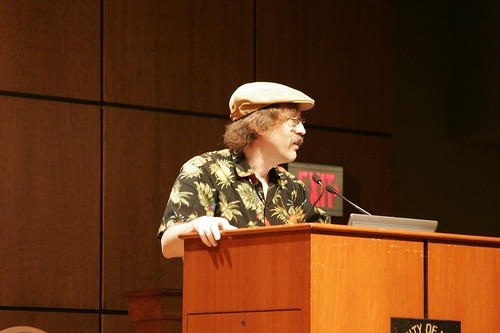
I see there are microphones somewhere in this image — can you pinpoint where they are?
[325,184,372,215]
[300,173,324,223]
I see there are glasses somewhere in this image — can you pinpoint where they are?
[288,116,305,127]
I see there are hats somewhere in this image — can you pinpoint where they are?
[229,82,315,122]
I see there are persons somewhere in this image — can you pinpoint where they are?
[157,83,331,262]
